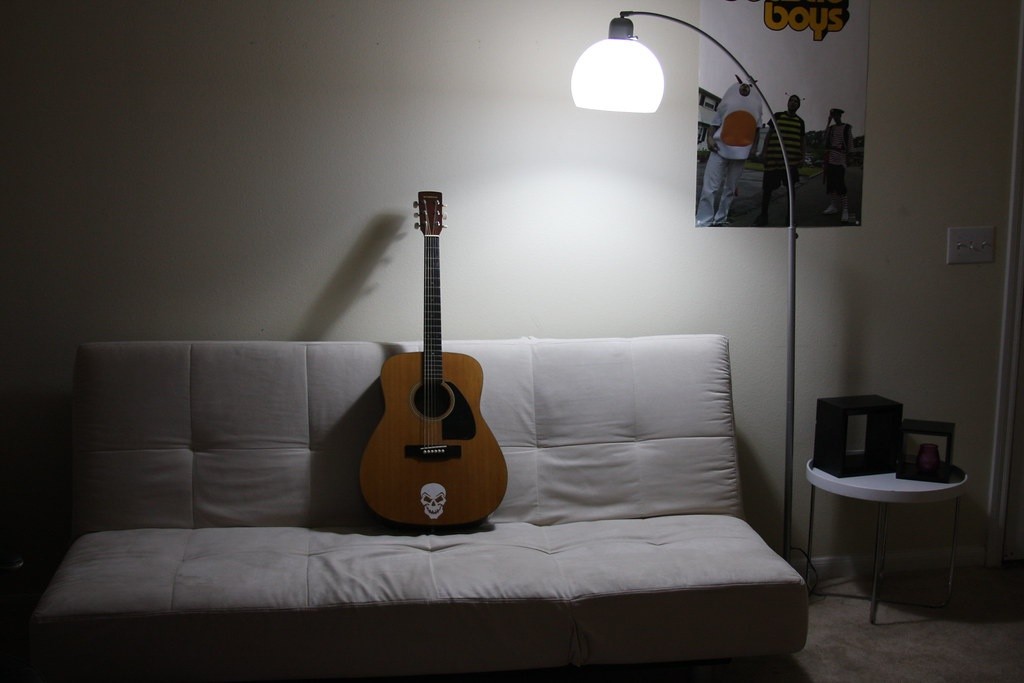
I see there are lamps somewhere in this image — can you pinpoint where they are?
[568,8,798,597]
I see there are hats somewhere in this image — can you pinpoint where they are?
[830,108,845,115]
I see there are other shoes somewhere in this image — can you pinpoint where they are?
[841,211,849,221]
[823,204,838,214]
[751,214,768,227]
[785,218,797,227]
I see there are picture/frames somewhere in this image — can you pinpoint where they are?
[895,418,955,484]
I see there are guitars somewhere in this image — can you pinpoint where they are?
[354,189,511,530]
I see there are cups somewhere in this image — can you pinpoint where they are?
[916,444,940,473]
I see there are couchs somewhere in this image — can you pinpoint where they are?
[28,340,811,683]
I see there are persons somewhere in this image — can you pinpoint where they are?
[696,74,856,227]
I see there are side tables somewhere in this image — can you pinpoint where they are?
[805,449,968,624]
